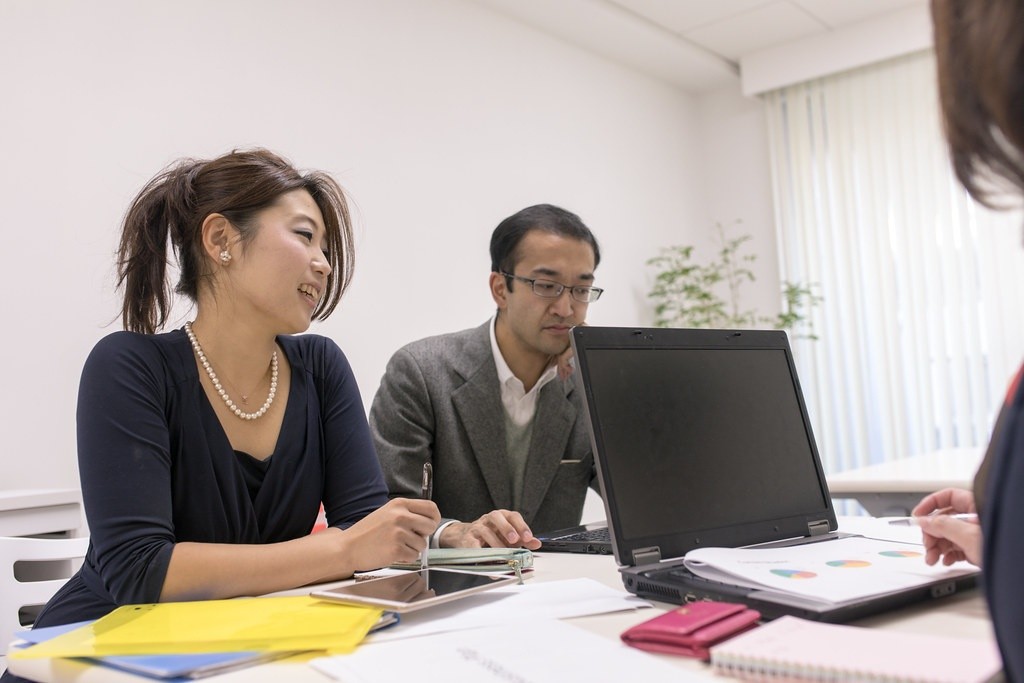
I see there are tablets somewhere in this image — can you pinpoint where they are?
[308,567,518,612]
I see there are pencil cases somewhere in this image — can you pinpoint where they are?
[390,548,534,586]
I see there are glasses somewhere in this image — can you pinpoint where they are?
[499,272,604,304]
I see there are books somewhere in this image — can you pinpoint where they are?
[710,615,1006,683]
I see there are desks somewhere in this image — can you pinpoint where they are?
[6,514,1006,683]
[823,446,988,517]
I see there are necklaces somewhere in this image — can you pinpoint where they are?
[185,321,279,421]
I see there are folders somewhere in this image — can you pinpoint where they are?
[16,595,400,683]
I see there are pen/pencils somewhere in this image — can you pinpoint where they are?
[421,463,433,570]
[874,513,982,527]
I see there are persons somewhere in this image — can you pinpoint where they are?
[0,151,441,683]
[910,0,1024,683]
[368,204,603,551]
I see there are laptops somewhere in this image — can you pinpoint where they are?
[521,520,613,556]
[567,325,983,625]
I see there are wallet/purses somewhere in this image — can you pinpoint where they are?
[620,599,765,661]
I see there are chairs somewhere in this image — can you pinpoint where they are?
[1,536,91,658]
